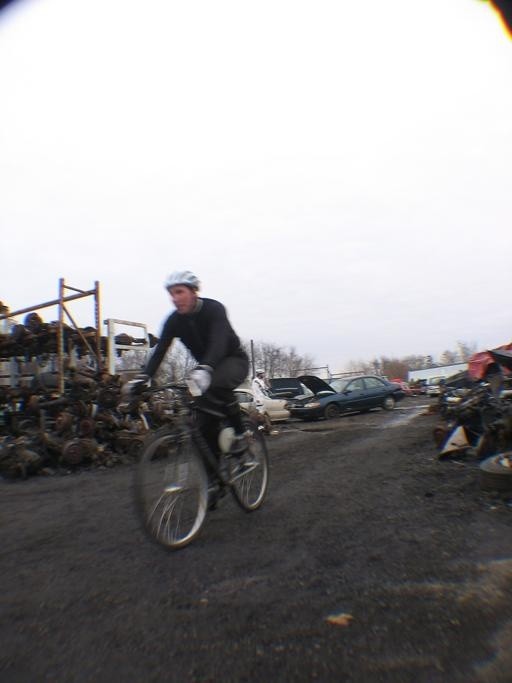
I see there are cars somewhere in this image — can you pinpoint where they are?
[232,385,290,426]
[388,373,451,398]
[268,374,313,400]
[281,372,404,419]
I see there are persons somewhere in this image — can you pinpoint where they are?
[251,369,272,399]
[121,270,250,511]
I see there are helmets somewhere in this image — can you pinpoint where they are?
[165,269,199,291]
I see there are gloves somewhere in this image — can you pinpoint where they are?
[120,373,152,395]
[188,365,215,393]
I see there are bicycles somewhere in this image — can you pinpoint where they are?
[128,382,271,552]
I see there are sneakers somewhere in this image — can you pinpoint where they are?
[221,434,249,455]
